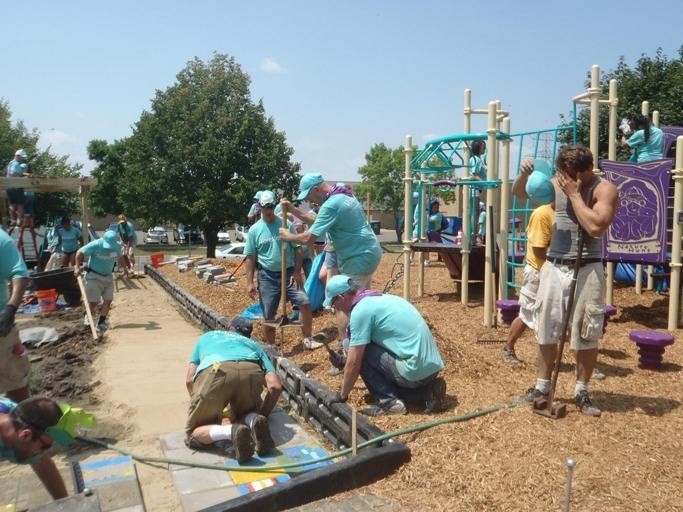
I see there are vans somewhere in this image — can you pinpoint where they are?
[172,221,204,245]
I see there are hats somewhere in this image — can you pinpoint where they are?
[118,213,126,223]
[322,274,356,307]
[259,191,276,207]
[525,159,557,206]
[15,149,28,158]
[297,174,324,200]
[253,191,262,199]
[102,230,117,249]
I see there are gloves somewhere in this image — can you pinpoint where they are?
[324,345,348,373]
[0,304,17,337]
[323,391,348,408]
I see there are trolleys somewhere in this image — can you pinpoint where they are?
[7,267,81,305]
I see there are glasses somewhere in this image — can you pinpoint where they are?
[331,297,338,307]
[260,205,276,209]
[34,432,51,450]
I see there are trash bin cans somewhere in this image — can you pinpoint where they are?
[369,221,381,235]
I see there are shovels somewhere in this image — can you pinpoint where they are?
[226,255,247,282]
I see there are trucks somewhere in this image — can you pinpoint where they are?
[5,219,101,268]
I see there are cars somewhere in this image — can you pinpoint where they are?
[104,222,116,232]
[141,227,168,244]
[216,243,246,261]
[234,225,249,243]
[216,227,229,244]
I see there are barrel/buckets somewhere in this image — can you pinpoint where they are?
[151,252,164,269]
[34,288,58,312]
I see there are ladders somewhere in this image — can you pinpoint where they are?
[8,214,40,260]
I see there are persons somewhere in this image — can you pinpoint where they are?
[622,113,665,164]
[500,200,606,379]
[184,315,282,465]
[511,145,619,417]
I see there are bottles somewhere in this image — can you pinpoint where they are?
[470,232,485,245]
[456,231,461,245]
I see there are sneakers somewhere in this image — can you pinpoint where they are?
[251,414,274,457]
[423,377,446,413]
[499,346,523,366]
[301,337,324,349]
[96,324,108,331]
[574,390,601,417]
[510,388,550,405]
[589,370,606,380]
[232,423,254,466]
[83,317,90,325]
[359,397,408,418]
